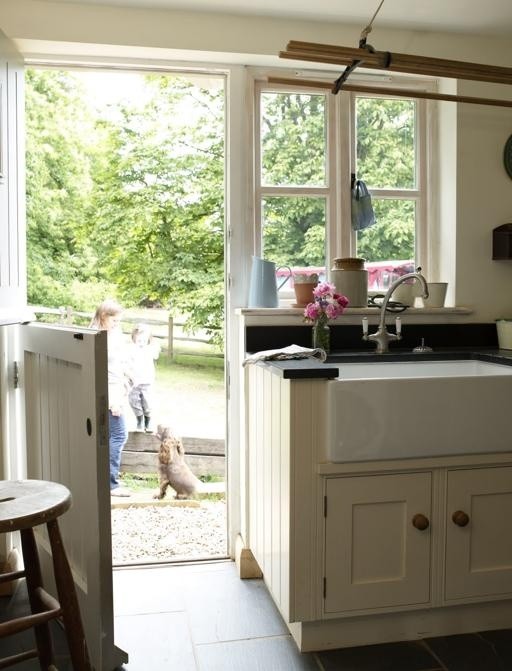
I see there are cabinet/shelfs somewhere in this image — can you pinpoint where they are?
[317,454,512,624]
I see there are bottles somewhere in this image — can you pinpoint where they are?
[312,317,331,354]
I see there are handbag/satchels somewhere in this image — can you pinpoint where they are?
[351,194,377,230]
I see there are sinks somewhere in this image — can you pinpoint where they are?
[322,359,512,463]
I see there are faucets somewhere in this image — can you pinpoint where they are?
[378,271,429,328]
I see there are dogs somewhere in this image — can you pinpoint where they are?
[153,424,203,501]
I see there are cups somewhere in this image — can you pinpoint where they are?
[391,283,416,308]
[421,282,448,309]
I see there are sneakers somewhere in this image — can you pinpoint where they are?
[110,482,130,497]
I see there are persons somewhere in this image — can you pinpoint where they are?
[87,298,136,497]
[124,327,162,433]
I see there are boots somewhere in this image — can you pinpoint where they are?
[136,415,153,433]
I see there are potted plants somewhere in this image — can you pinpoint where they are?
[293,273,320,305]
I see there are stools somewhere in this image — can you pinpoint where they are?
[0,477,90,671]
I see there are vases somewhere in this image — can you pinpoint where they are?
[330,256,369,308]
[310,321,331,355]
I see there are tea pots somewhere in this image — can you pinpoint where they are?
[248,256,292,307]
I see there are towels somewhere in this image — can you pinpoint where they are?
[240,339,328,367]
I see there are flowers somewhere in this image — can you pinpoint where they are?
[300,281,349,346]
